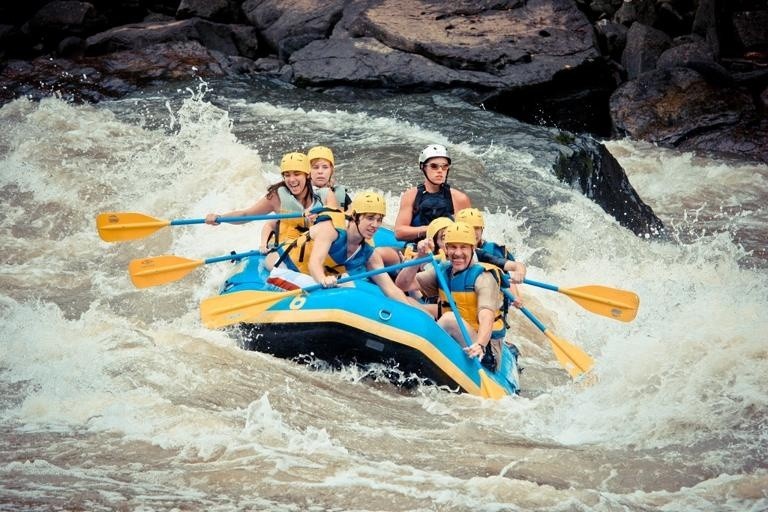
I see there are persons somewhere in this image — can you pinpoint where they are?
[452,207,524,309]
[264,190,412,308]
[367,142,474,307]
[396,221,501,374]
[258,144,353,256]
[424,216,527,284]
[203,152,338,249]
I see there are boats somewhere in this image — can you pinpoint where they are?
[215,225,522,406]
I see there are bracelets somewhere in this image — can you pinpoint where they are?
[478,342,487,353]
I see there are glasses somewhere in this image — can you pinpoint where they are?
[424,162,450,171]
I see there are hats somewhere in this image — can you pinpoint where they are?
[419,144,451,165]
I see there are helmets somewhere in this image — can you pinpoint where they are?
[426,217,455,240]
[445,221,476,245]
[456,208,484,228]
[307,146,334,167]
[352,192,386,217]
[280,153,310,174]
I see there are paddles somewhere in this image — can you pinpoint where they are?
[126,253,272,289]
[523,279,640,321]
[95,206,326,242]
[197,249,445,329]
[424,245,506,400]
[499,285,593,380]
